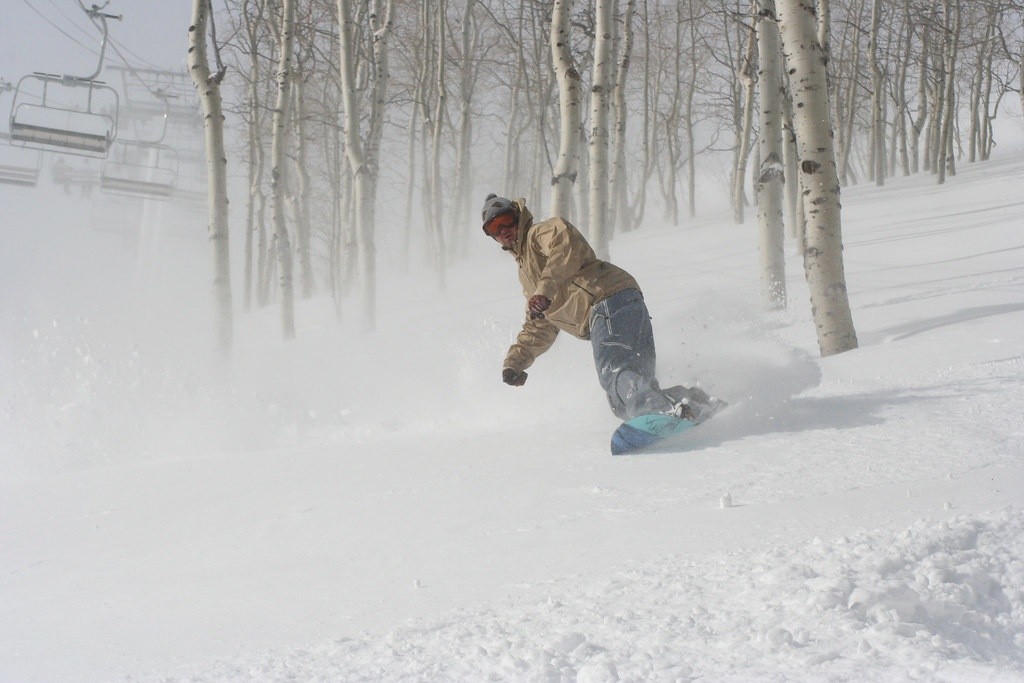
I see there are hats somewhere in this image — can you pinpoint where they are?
[481,193,514,226]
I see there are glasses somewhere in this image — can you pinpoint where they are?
[483,210,517,236]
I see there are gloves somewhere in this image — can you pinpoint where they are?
[502,368,528,386]
[528,295,552,320]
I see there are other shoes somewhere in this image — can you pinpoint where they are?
[690,386,717,402]
[664,400,695,420]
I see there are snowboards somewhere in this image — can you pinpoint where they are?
[610,399,729,456]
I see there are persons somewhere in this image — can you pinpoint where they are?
[482,193,710,421]
